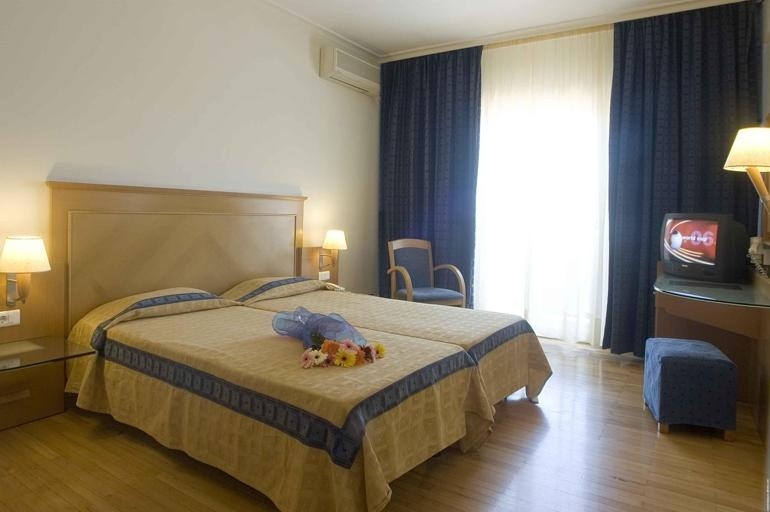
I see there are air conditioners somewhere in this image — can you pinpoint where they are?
[318,44,383,99]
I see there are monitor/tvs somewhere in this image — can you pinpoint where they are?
[660,211,751,285]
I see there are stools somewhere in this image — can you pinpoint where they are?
[643,334,741,444]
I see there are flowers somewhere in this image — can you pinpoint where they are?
[299,338,386,371]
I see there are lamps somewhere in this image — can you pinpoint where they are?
[0,234,52,307]
[318,229,347,272]
[721,126,768,220]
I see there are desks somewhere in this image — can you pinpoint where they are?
[648,260,768,446]
[0,335,97,375]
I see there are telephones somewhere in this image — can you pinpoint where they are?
[325,283,345,292]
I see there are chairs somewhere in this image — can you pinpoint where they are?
[389,239,466,308]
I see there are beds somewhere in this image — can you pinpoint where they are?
[45,181,541,512]
